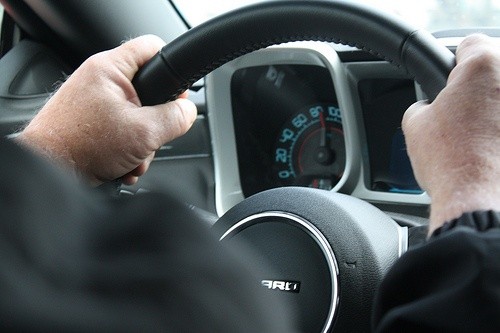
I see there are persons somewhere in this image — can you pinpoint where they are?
[1,32,500,333]
